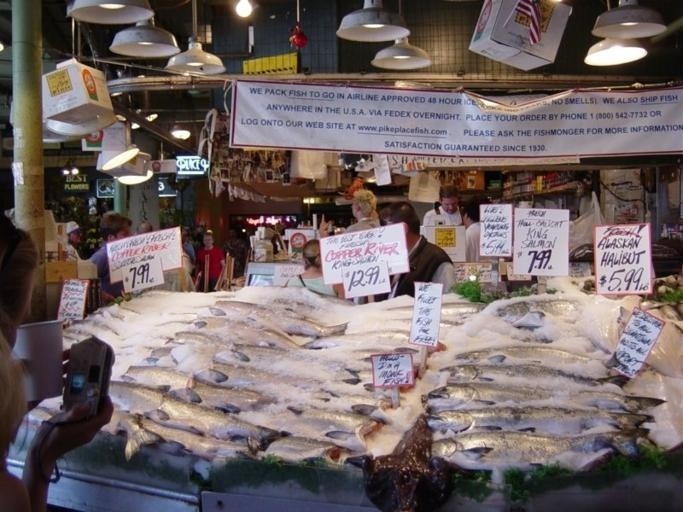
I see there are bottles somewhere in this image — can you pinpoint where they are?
[56,222,68,262]
[543,171,569,189]
[13,321,61,402]
[253,238,274,264]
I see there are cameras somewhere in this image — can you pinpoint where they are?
[62,340,114,415]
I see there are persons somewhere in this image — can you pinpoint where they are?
[460,194,498,263]
[285,240,344,299]
[346,189,380,233]
[66,213,249,306]
[0,214,113,512]
[423,186,463,226]
[364,202,455,304]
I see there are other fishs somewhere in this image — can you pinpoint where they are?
[56,295,668,472]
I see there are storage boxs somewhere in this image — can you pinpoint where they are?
[468,0,573,72]
[30,261,79,322]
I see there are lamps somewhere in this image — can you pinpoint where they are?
[335,0,411,42]
[584,1,649,67]
[107,16,181,58]
[371,0,432,71]
[72,0,156,25]
[591,0,666,39]
[164,0,226,75]
[43,61,154,186]
